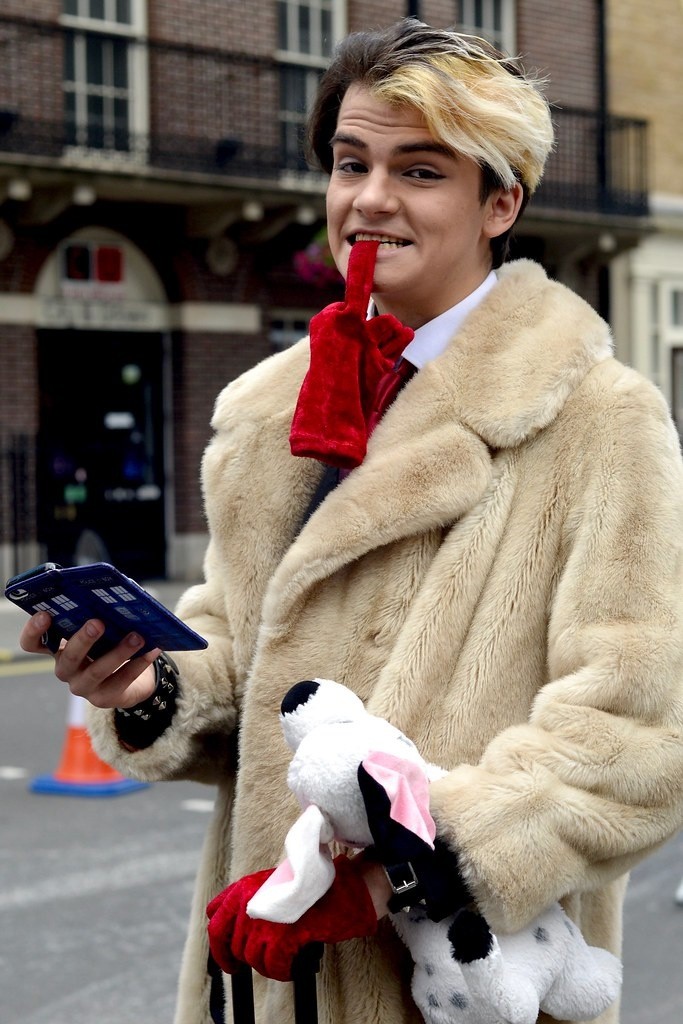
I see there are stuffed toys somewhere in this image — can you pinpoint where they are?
[244,680,625,1024]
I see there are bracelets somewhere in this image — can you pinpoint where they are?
[116,652,180,721]
[382,853,426,914]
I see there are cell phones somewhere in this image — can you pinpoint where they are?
[3,562,209,663]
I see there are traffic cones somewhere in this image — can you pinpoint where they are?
[27,692,149,797]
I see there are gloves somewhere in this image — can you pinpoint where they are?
[207,854,378,982]
[290,240,415,469]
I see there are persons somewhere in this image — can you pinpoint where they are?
[20,23,682,1024]
